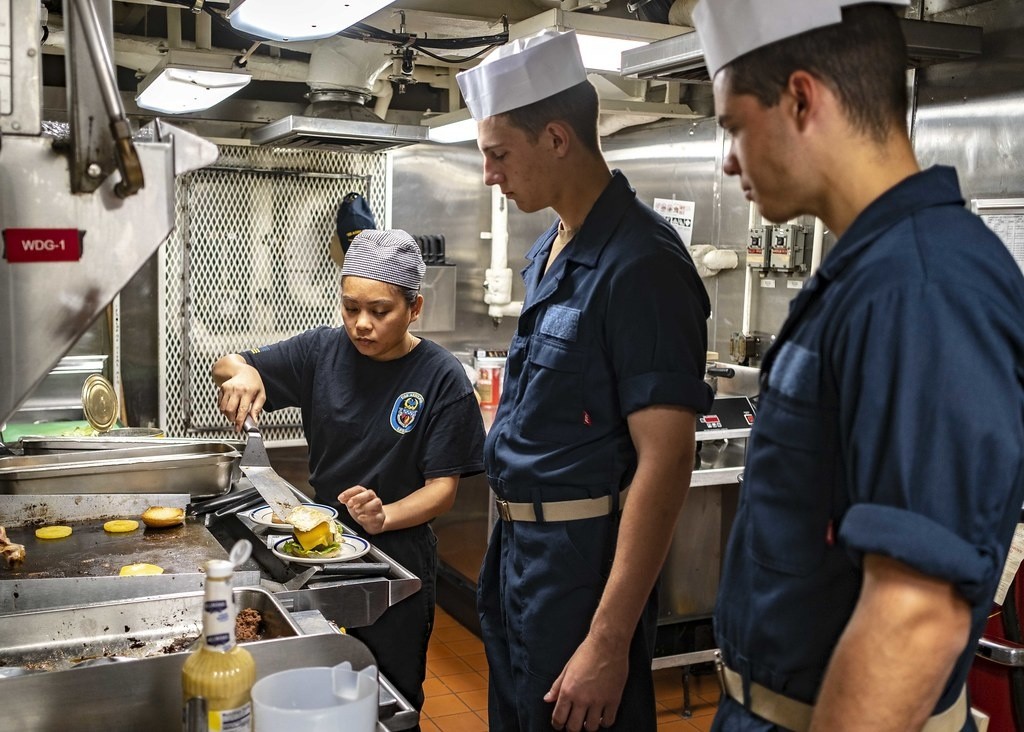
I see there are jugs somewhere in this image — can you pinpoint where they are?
[251,661,380,732]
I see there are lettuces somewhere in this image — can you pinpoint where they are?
[282,523,343,555]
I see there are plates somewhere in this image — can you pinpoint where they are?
[248,503,338,528]
[271,534,370,563]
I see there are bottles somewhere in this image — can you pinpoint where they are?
[181,539,255,732]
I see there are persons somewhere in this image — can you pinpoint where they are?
[693,0,1024,732]
[452,23,712,732]
[212,228,486,732]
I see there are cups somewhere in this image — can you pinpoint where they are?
[476,357,506,409]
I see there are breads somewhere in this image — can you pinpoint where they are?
[292,548,341,558]
[141,506,185,528]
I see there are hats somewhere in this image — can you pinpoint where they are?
[340,227,426,290]
[691,0,912,79]
[454,27,588,119]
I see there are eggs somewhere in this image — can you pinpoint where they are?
[287,506,339,534]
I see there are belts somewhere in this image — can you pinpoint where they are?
[496,485,628,522]
[714,651,967,731]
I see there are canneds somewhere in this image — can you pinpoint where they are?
[81,374,165,437]
[476,357,508,411]
